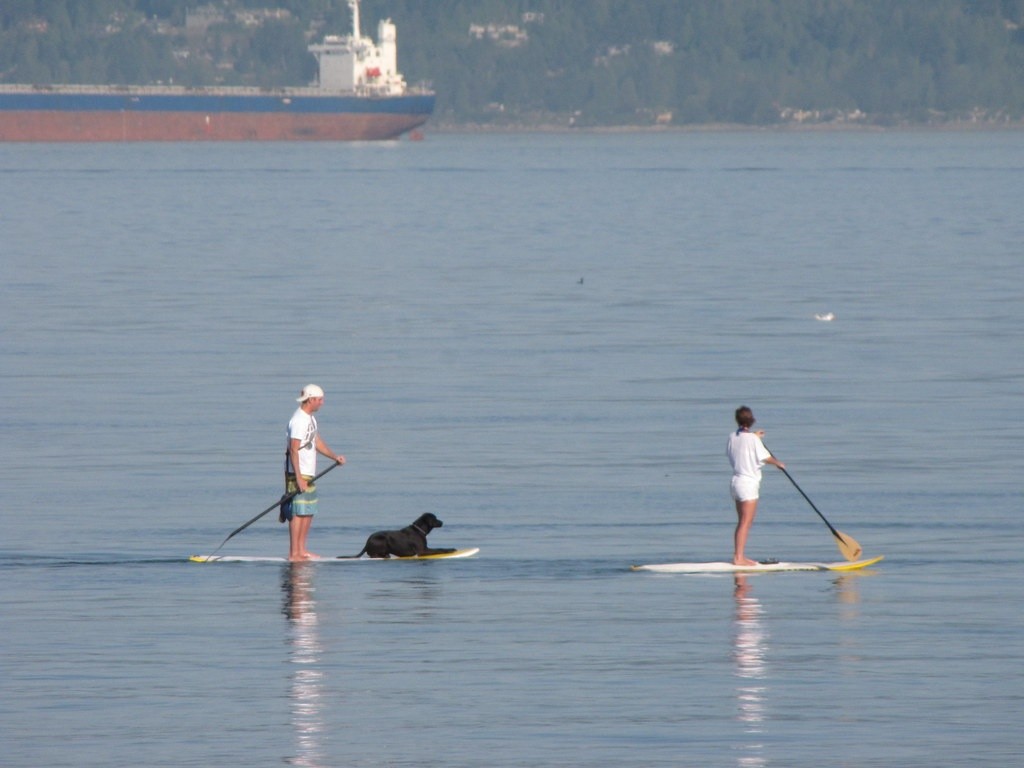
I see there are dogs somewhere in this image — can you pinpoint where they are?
[337,513,457,558]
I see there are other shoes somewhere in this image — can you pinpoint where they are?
[759,557,779,564]
[278,495,293,523]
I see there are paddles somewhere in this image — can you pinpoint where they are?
[762,441,862,562]
[210,459,342,555]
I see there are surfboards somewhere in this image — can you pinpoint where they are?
[631,554,885,573]
[189,548,480,562]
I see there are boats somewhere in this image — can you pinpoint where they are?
[0,0,437,142]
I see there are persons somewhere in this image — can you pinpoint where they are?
[283,384,346,561]
[726,405,785,566]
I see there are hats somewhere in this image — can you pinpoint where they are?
[296,383,324,402]
